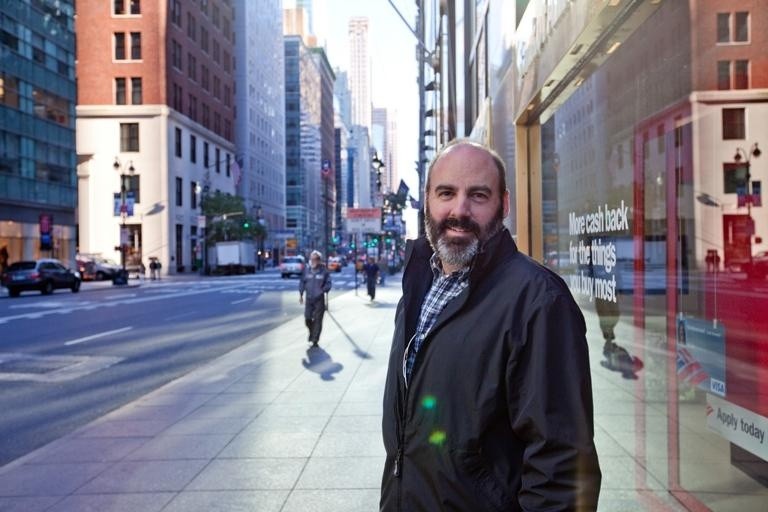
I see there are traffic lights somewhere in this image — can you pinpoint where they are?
[242,216,249,229]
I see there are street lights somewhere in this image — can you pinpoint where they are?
[370,150,385,254]
[733,139,761,215]
[112,153,136,269]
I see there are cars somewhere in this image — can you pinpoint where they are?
[86,253,122,282]
[727,250,767,280]
[0,256,86,298]
[75,254,95,273]
[327,247,402,275]
[543,250,572,272]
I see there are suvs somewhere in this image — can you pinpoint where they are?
[279,255,306,279]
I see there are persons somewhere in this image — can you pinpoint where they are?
[137,257,163,280]
[376,139,603,512]
[589,266,643,341]
[298,250,332,348]
[361,257,379,302]
